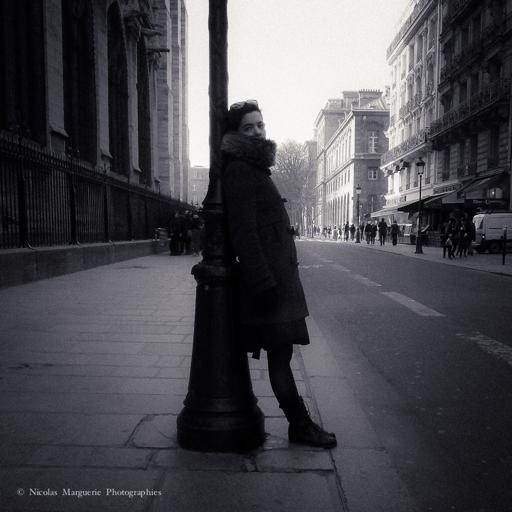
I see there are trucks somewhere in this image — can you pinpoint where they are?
[472,212,512,254]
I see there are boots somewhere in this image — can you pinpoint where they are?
[279,393,338,450]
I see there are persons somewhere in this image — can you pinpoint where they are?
[314,218,429,246]
[169,209,205,255]
[440,216,476,260]
[219,103,337,450]
[292,226,296,236]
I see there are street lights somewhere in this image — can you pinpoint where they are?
[355,184,361,243]
[415,157,425,254]
